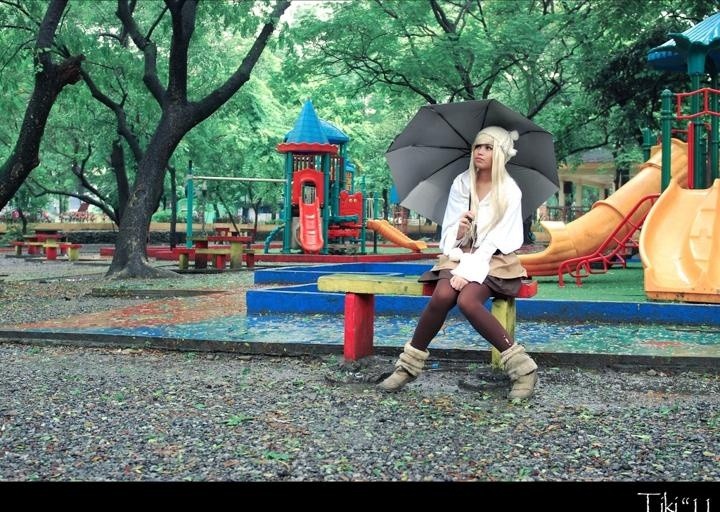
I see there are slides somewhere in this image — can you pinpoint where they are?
[517,139,689,275]
[298,196,324,254]
[638,177,720,304]
[367,220,426,253]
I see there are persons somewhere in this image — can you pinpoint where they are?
[376,127,539,401]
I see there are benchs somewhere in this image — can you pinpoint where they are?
[171,228,259,272]
[9,228,84,262]
[316,271,538,368]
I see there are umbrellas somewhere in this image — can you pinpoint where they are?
[385,99,561,228]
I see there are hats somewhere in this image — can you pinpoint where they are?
[477,126,519,165]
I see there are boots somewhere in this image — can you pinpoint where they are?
[499,342,538,401]
[378,343,429,393]
